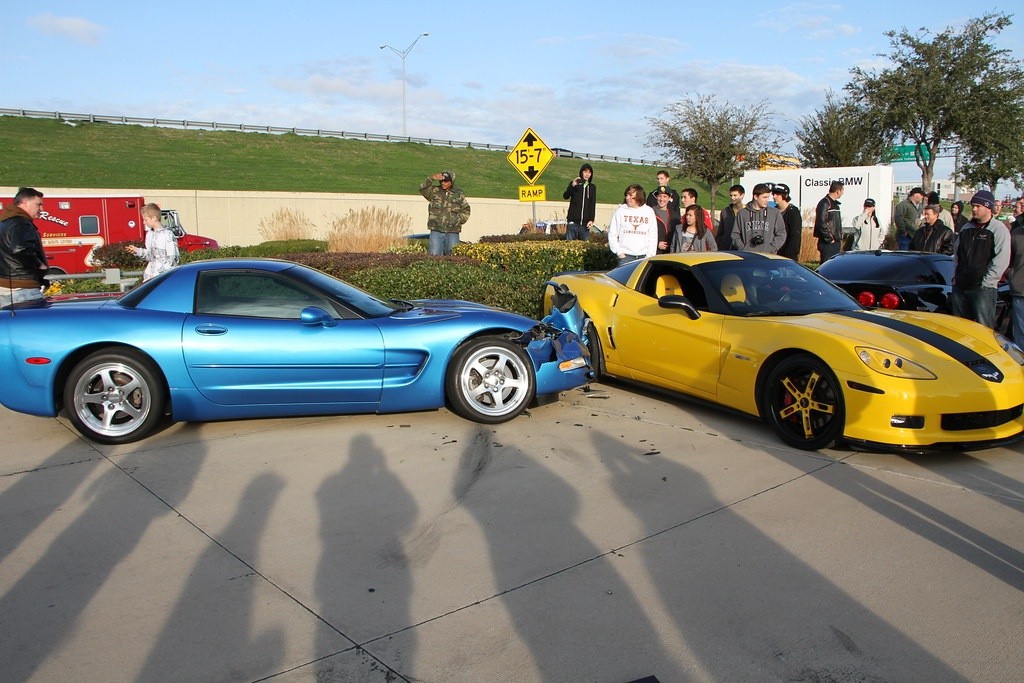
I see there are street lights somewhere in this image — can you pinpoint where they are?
[379,33,429,136]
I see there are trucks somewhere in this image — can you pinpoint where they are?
[0,195,219,283]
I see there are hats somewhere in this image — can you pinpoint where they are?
[654,186,671,197]
[442,171,452,181]
[864,198,875,206]
[971,190,994,211]
[771,183,789,194]
[927,191,939,204]
[910,187,926,196]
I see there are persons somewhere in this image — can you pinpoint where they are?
[851,199,886,250]
[652,186,673,255]
[994,191,1024,352]
[951,190,1011,330]
[770,184,802,262]
[646,170,681,224]
[125,203,180,282]
[563,164,597,240]
[952,201,969,232]
[895,188,926,250]
[419,170,470,256]
[670,205,718,252]
[0,187,50,309]
[813,181,844,265]
[715,184,746,250]
[919,192,954,233]
[608,184,658,265]
[727,184,787,255]
[907,205,954,256]
[681,188,712,231]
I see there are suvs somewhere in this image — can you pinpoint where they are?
[520,222,604,235]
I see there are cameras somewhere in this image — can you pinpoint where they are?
[751,235,764,245]
[578,179,585,183]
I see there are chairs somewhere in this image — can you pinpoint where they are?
[655,274,684,299]
[720,273,746,303]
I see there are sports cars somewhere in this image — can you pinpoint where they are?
[545,253,1023,452]
[0,256,593,446]
[779,250,1012,335]
